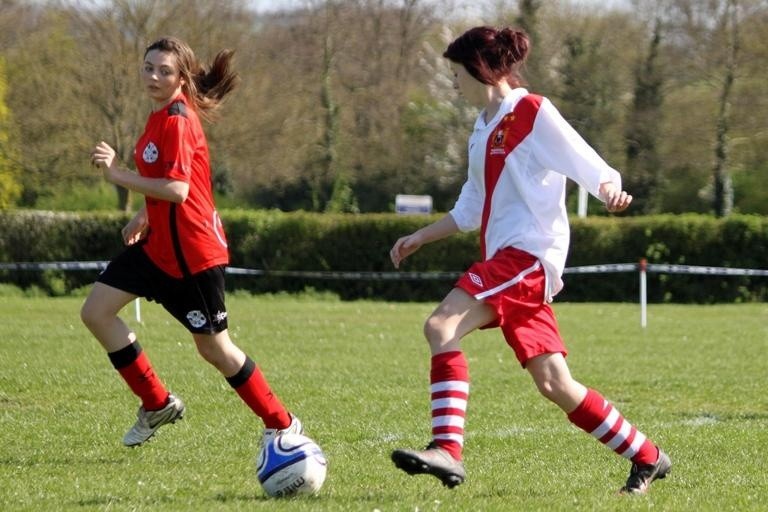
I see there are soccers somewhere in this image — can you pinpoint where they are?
[256,435,328,499]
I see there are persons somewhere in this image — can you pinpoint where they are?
[76,35,304,456]
[386,23,676,497]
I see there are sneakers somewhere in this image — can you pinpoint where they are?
[391,442,465,488]
[122,392,186,450]
[258,411,304,454]
[615,445,671,499]
